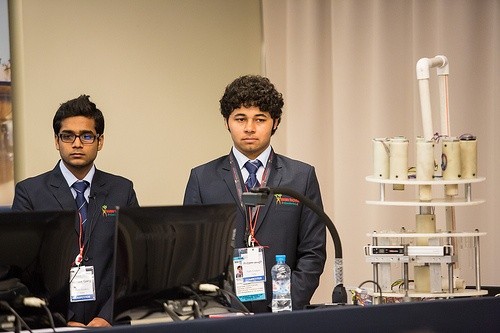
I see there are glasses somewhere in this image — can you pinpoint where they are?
[57,130,100,144]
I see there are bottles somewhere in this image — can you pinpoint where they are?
[271,255,292,313]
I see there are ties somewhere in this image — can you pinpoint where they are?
[71,180,90,254]
[244,160,263,225]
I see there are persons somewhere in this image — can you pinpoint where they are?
[182,74,329,314]
[9,95,140,329]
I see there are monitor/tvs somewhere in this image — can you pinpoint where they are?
[111,202,236,323]
[0,212,79,330]
